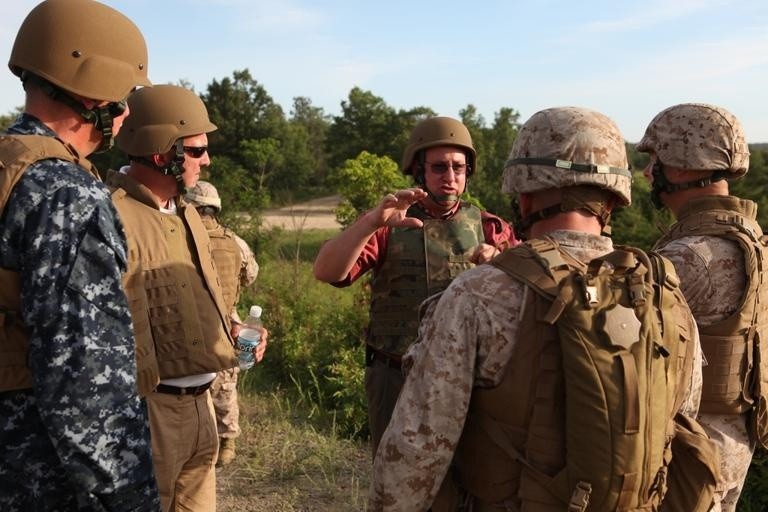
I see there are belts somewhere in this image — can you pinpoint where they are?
[154,383,213,396]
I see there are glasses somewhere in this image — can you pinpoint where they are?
[424,161,471,175]
[171,145,208,159]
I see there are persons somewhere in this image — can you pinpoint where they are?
[365,107,704,510]
[0,1,160,510]
[105,85,268,511]
[183,182,260,466]
[635,104,768,510]
[313,117,522,464]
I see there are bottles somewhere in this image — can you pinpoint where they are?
[236,303,262,372]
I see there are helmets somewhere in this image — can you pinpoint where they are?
[402,117,476,176]
[637,103,750,180]
[116,85,219,155]
[504,107,632,207]
[8,0,153,102]
[185,181,222,213]
